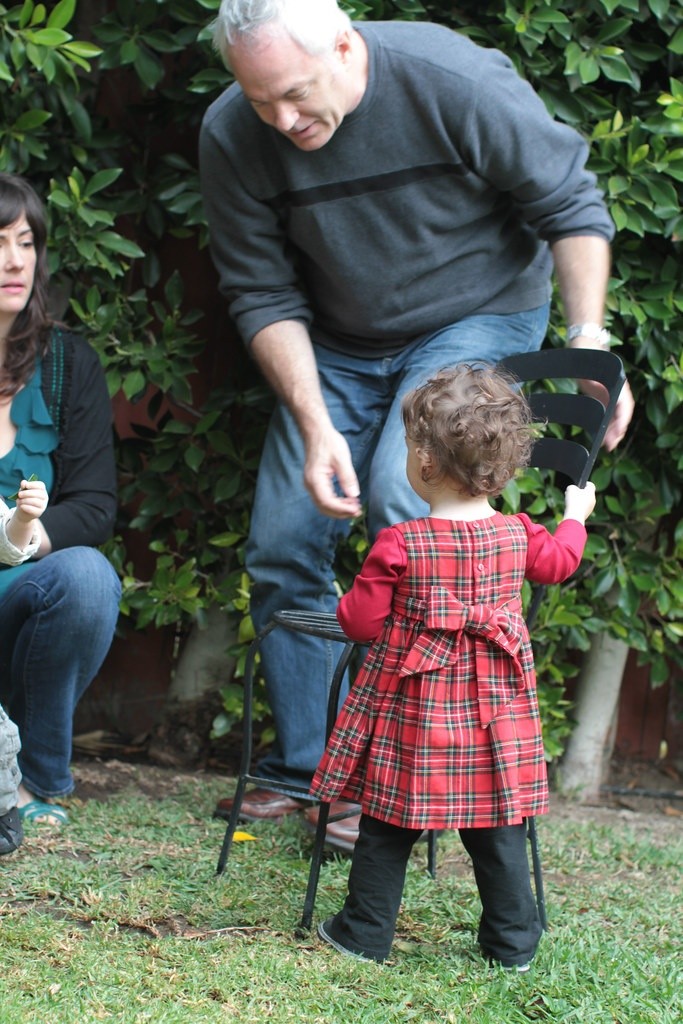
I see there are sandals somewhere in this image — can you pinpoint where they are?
[15,797,69,827]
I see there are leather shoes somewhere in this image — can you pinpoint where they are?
[213,788,313,824]
[301,800,362,853]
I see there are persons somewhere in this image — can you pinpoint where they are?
[0,479,48,854]
[199,0,636,848]
[306,365,596,971]
[0,172,125,823]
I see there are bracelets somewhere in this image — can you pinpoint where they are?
[567,322,609,347]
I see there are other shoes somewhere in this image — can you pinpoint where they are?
[317,918,382,965]
[481,949,531,975]
[0,806,22,854]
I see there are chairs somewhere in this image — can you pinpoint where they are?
[215,347,626,942]
